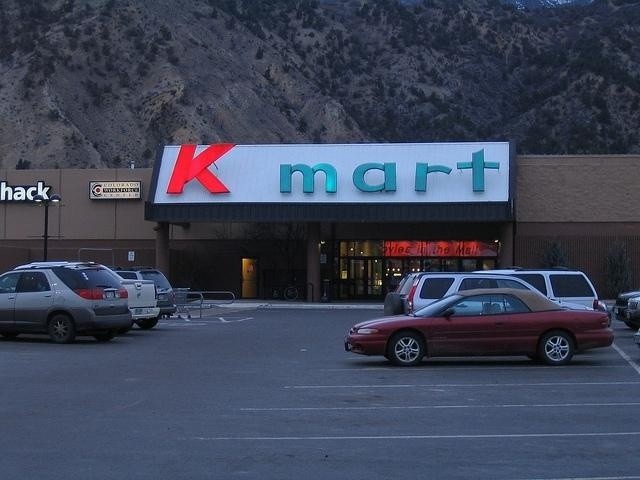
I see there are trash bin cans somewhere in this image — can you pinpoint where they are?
[321,279,332,304]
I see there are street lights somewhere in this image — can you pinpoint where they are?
[33,194,62,262]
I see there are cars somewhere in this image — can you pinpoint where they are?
[613,289,640,344]
[0,261,177,344]
[344,266,614,367]
